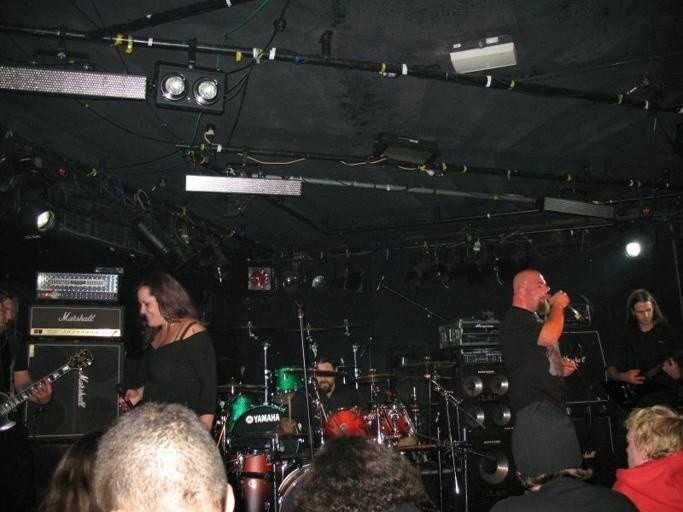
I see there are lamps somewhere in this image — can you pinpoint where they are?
[13,194,57,238]
[151,47,227,115]
[132,211,209,272]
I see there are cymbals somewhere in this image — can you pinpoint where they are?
[351,374,401,383]
[399,361,457,373]
[284,369,346,377]
[218,383,266,395]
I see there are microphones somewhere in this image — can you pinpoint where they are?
[565,304,585,320]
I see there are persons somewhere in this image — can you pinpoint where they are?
[305,357,379,420]
[96,399,239,511]
[274,434,434,512]
[35,434,99,512]
[119,272,220,432]
[606,288,680,399]
[2,290,53,511]
[613,406,682,512]
[484,401,637,512]
[498,269,577,417]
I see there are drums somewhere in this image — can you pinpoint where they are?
[231,403,300,464]
[225,445,275,511]
[321,404,369,437]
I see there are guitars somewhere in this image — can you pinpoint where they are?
[0,347,96,431]
[614,349,683,411]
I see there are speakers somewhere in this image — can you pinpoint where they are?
[22,340,122,440]
[447,364,522,504]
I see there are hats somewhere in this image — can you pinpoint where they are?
[511,402,583,476]
